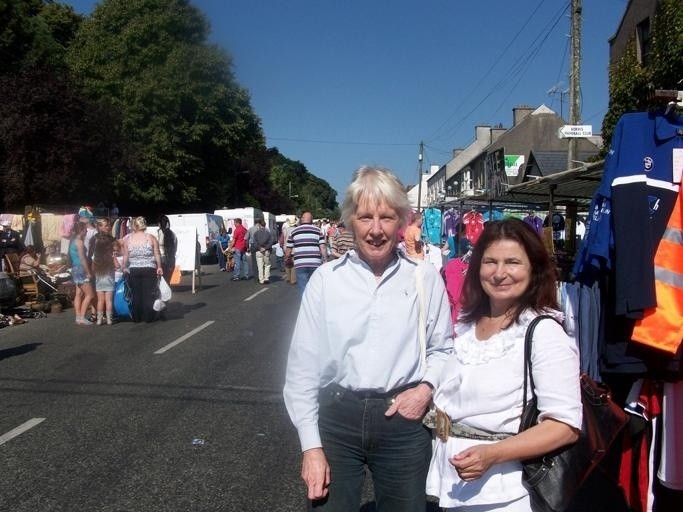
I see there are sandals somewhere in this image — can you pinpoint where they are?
[88,314,97,322]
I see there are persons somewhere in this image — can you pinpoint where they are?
[19,244,58,284]
[426,217,585,512]
[216,167,424,326]
[67,216,177,326]
[282,169,453,512]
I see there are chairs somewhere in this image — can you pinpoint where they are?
[5,252,42,300]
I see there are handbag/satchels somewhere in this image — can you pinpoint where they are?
[513,313,631,512]
[157,274,172,301]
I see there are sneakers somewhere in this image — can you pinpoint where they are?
[75,318,80,323]
[219,266,297,285]
[79,319,93,325]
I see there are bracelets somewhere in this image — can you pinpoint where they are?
[419,381,436,399]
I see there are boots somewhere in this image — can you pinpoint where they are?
[95,313,103,325]
[105,312,112,325]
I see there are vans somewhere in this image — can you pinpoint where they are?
[162,211,233,265]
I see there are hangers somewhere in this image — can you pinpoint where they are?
[423,204,478,222]
[663,78,683,121]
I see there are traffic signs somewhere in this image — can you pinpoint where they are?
[559,124,592,138]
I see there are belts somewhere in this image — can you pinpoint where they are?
[419,408,513,442]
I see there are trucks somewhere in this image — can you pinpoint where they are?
[260,210,279,244]
[273,213,299,238]
[215,205,265,245]
